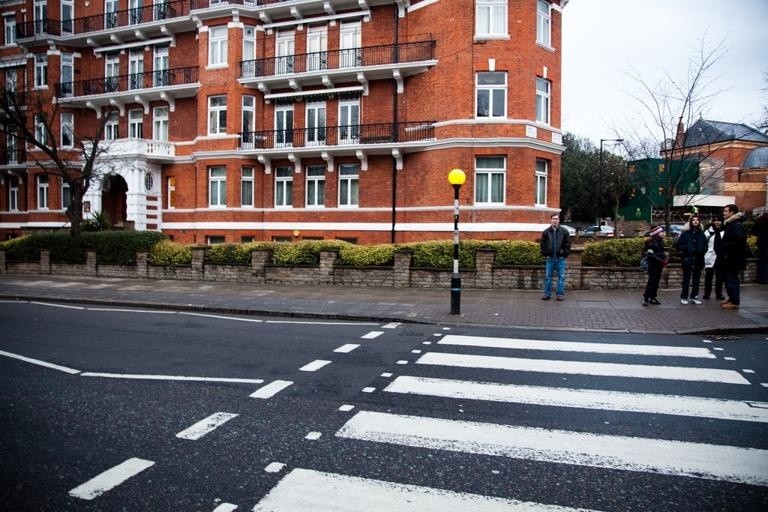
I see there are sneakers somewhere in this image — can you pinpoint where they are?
[642,298,661,306]
[680,298,688,305]
[557,296,564,301]
[719,299,739,310]
[541,295,551,300]
[703,294,725,300]
[690,296,702,305]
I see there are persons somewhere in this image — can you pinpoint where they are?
[675,215,708,305]
[705,217,725,300]
[642,224,668,305]
[714,203,748,311]
[541,213,572,302]
[752,211,768,284]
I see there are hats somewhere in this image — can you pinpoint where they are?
[648,224,664,237]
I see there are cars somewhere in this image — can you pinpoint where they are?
[578,226,625,238]
[558,224,577,236]
[647,224,686,237]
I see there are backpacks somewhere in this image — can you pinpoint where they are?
[640,249,654,273]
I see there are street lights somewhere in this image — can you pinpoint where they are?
[447,167,467,316]
[598,137,624,232]
